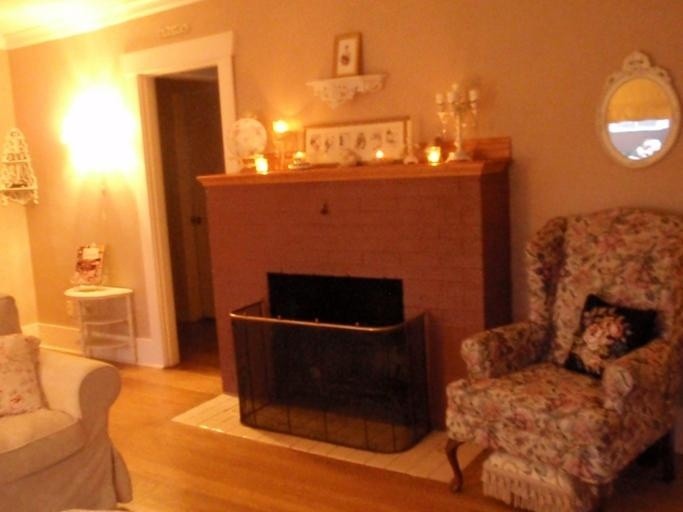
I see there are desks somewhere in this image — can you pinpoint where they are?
[64,283,137,367]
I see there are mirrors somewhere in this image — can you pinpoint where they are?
[598,48,683,172]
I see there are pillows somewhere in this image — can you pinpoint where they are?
[560,290,661,382]
[1,334,46,415]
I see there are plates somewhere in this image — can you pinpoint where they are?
[228,117,268,162]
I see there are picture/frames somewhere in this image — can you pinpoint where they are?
[333,32,363,77]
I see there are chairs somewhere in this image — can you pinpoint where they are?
[1,295,138,511]
[441,201,683,512]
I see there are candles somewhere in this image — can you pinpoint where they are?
[435,83,480,108]
